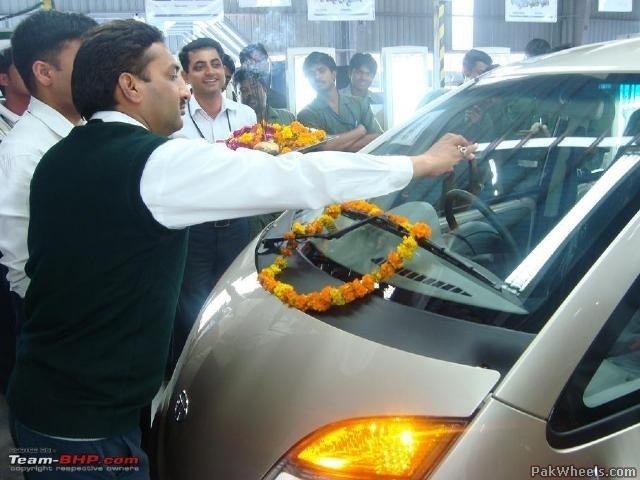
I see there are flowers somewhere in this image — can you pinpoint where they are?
[257,198,430,316]
[224,120,330,154]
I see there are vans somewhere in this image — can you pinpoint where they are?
[151,31,640,480]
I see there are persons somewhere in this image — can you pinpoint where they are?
[0,8,100,358]
[0,47,31,143]
[223,42,385,153]
[7,20,480,480]
[166,37,258,337]
[415,40,605,211]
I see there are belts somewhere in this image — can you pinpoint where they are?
[203,218,238,227]
